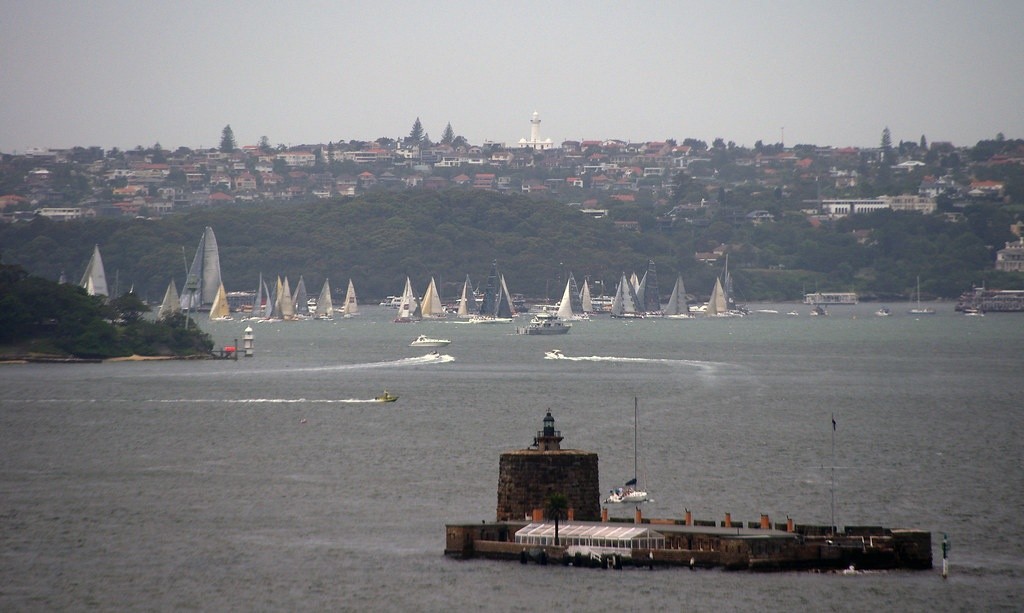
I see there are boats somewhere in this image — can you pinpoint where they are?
[379,296,403,309]
[810,308,830,316]
[409,335,451,347]
[520,313,572,335]
[375,389,399,402]
[876,277,1024,317]
[426,350,440,359]
[468,315,512,323]
[803,293,859,305]
[787,311,799,316]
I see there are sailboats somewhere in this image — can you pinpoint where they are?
[395,276,447,323]
[208,281,234,321]
[604,397,647,502]
[556,273,614,322]
[704,277,744,318]
[179,226,221,312]
[610,260,696,320]
[241,272,361,323]
[457,259,518,324]
